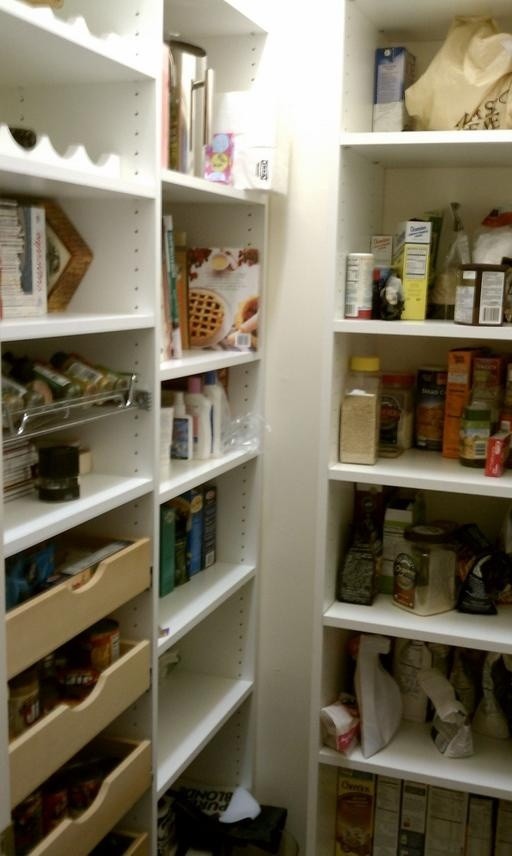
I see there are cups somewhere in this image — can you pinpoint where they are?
[170,38,212,183]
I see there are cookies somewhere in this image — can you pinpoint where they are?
[188,289,232,347]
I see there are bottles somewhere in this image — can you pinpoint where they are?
[339,353,383,465]
[2,348,129,431]
[390,524,461,617]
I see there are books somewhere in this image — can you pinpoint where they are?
[158,481,222,599]
[1,438,45,508]
[170,36,212,179]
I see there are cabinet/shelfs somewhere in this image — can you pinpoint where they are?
[295,5,511,856]
[1,0,295,856]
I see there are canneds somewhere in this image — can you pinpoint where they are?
[416,364,448,450]
[84,618,121,669]
